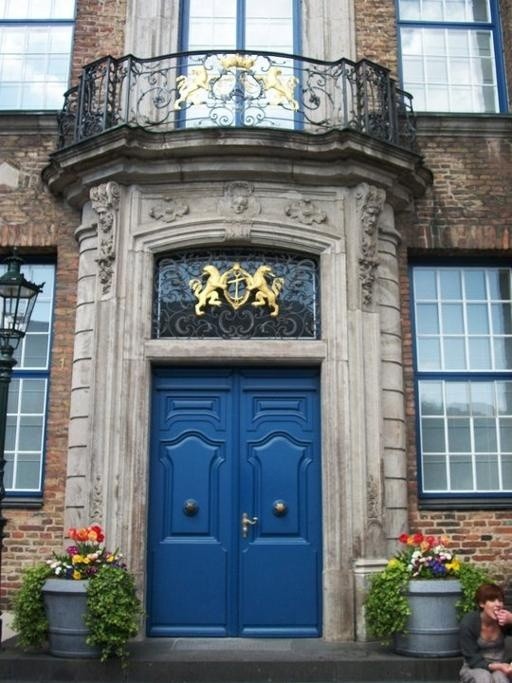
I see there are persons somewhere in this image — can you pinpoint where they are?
[458,583,512,683]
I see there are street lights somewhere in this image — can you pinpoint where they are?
[0,236,52,558]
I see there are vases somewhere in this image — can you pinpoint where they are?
[38,578,104,660]
[392,579,463,658]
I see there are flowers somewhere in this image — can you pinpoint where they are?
[6,525,152,673]
[361,532,499,649]
